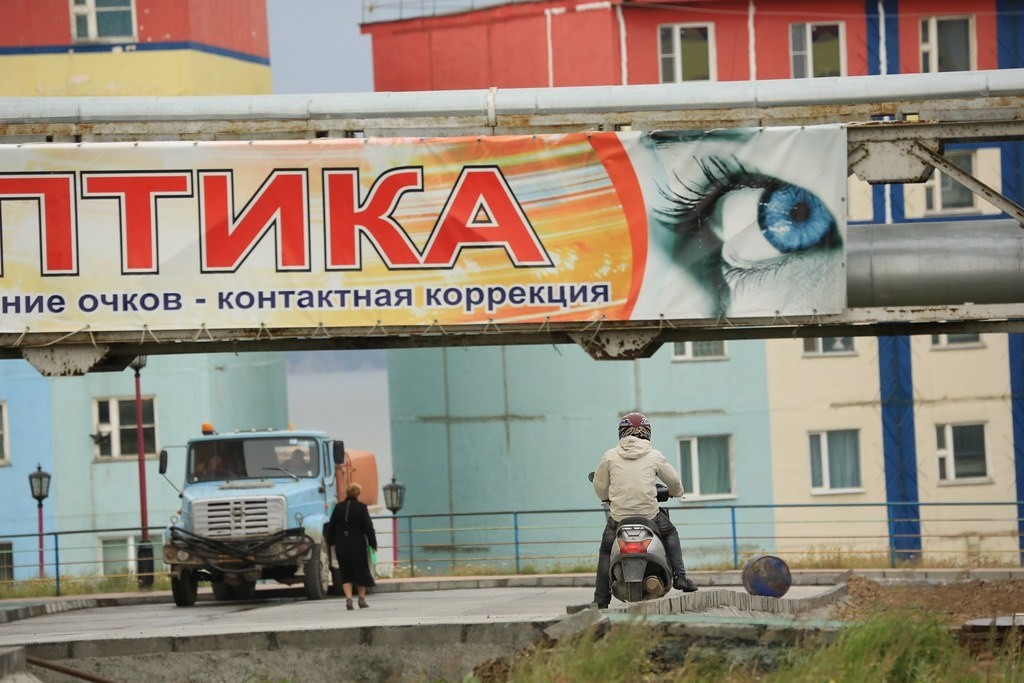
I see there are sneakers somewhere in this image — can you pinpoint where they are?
[673,574,699,593]
[591,602,609,609]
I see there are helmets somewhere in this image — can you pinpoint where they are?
[618,412,652,442]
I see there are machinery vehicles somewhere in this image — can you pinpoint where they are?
[157,421,381,607]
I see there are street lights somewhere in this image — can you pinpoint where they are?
[381,478,407,574]
[27,463,52,575]
[128,355,155,587]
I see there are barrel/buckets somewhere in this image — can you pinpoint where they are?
[741,555,792,598]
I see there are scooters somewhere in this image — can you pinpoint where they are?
[586,472,687,601]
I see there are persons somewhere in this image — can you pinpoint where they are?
[590,412,698,609]
[194,449,226,482]
[326,483,377,610]
[637,122,851,313]
[284,448,307,475]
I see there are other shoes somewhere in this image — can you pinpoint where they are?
[358,597,369,609]
[346,598,353,610]
